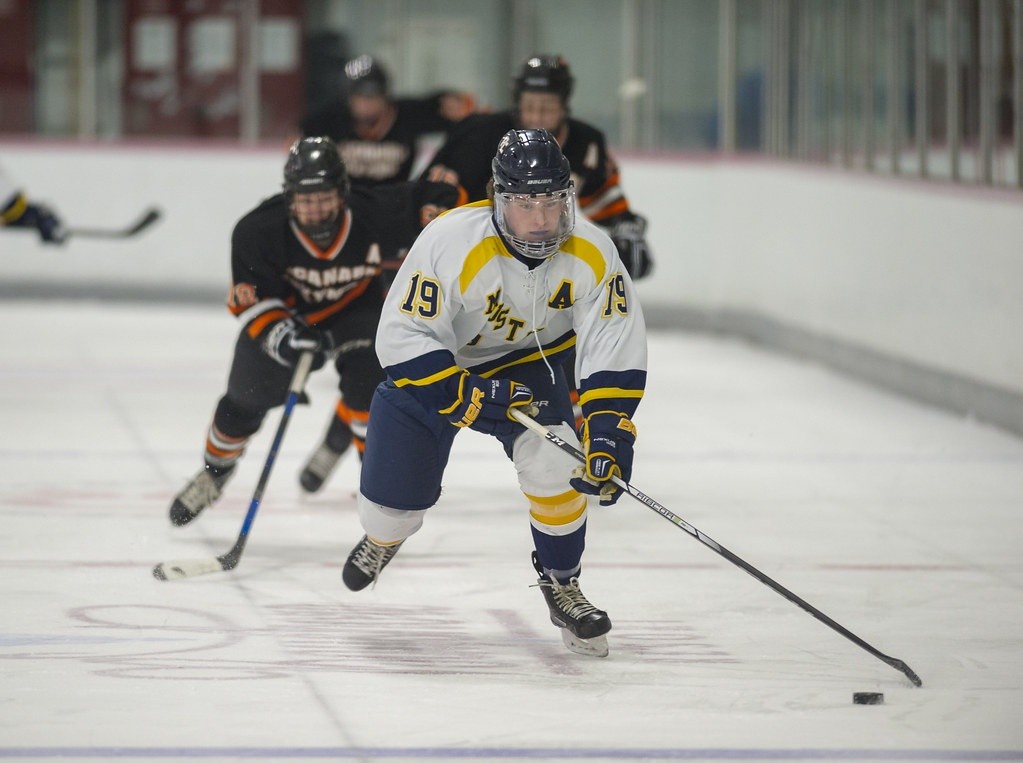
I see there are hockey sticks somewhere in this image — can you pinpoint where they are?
[507,406,928,691]
[66,209,160,239]
[152,350,316,583]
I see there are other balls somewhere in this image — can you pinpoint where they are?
[853,692,885,706]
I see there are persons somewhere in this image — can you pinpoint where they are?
[301,54,653,492]
[302,56,456,189]
[342,127,648,658]
[0,178,71,246]
[169,138,461,527]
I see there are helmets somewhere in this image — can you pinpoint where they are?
[492,128,571,197]
[284,137,346,195]
[514,54,572,103]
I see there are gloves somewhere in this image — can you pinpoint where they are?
[437,367,534,437]
[23,207,60,240]
[612,218,650,277]
[274,328,327,371]
[569,410,637,506]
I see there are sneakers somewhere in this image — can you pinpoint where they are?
[342,534,406,592]
[529,551,612,657]
[301,442,342,493]
[170,468,232,527]
